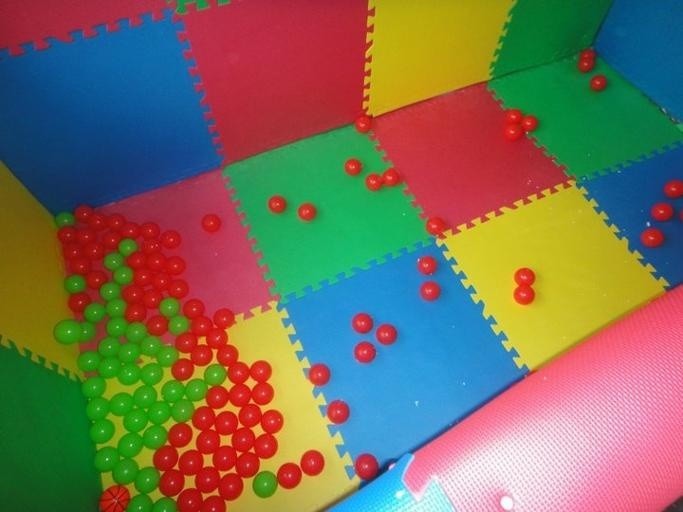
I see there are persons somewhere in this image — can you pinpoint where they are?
[81,376,106,398]
[162,380,185,403]
[109,392,132,416]
[134,384,157,409]
